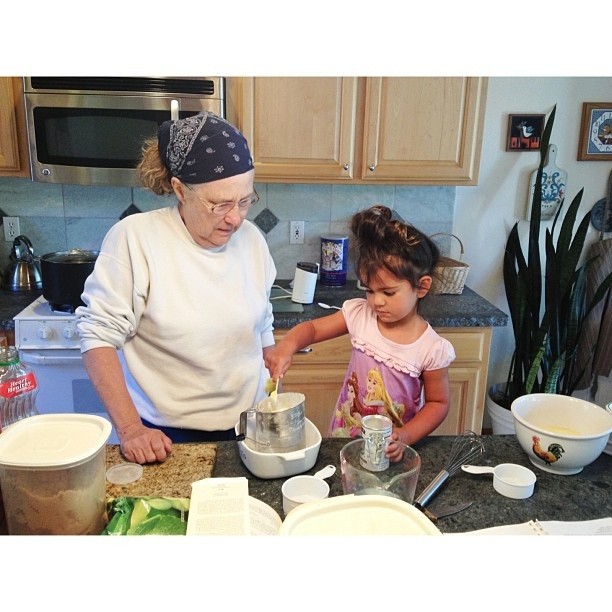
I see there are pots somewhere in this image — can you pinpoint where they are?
[32,249,100,304]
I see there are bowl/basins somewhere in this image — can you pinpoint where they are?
[233,414,322,478]
[510,393,612,476]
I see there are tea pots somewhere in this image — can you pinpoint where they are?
[340,438,421,504]
[0,234,43,292]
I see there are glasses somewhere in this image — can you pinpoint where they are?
[182,183,261,216]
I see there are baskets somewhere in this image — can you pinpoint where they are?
[430,232,470,295]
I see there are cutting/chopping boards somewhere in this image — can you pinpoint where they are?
[103,444,217,503]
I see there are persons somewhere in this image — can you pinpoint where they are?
[263,204,456,461]
[73,111,279,463]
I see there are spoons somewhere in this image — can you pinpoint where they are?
[318,302,341,310]
[270,378,280,408]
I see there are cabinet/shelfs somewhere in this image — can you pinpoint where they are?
[266,327,492,436]
[0,77,30,178]
[224,77,487,187]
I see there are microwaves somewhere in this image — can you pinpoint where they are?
[21,77,226,187]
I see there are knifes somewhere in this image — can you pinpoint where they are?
[423,500,475,523]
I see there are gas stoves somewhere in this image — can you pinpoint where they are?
[13,295,87,349]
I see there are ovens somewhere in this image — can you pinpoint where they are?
[15,347,123,444]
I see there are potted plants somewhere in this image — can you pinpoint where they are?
[487,98,611,437]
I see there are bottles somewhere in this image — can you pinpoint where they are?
[320,234,348,289]
[291,262,318,304]
[0,343,41,435]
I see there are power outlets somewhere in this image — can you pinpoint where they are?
[290,221,304,244]
[2,216,21,242]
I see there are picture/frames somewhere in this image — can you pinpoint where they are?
[505,114,545,152]
[576,102,611,160]
